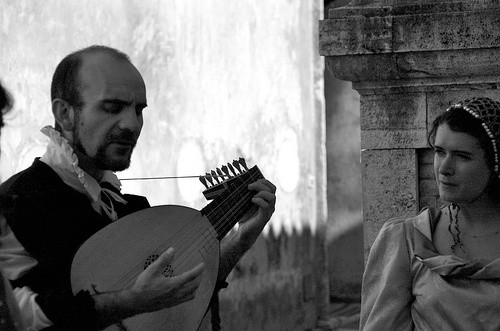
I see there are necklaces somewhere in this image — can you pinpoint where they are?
[462,231,500,238]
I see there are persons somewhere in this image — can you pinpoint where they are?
[0,83,25,331]
[0,45,276,331]
[359,97,500,331]
